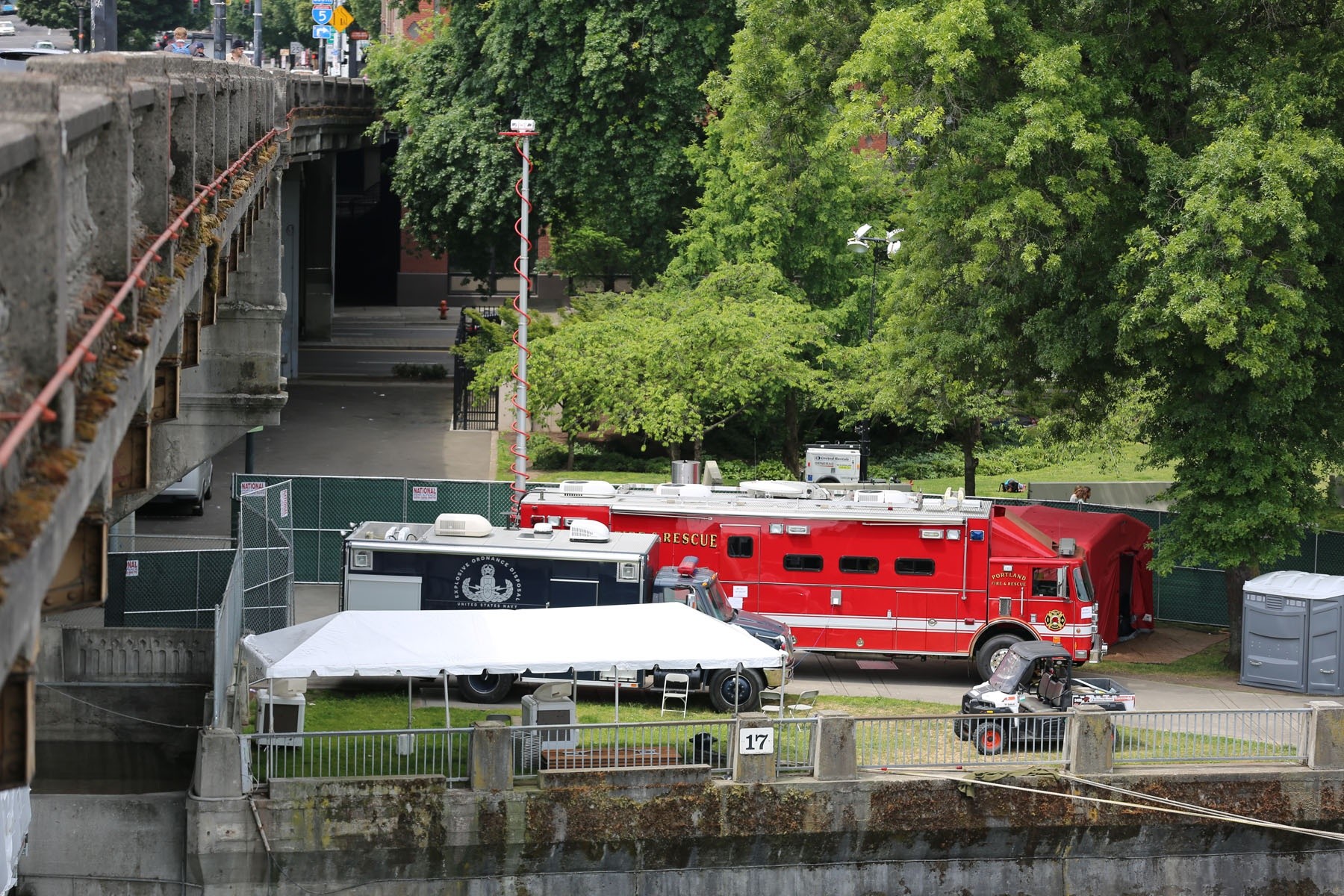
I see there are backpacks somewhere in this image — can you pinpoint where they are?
[171,43,191,55]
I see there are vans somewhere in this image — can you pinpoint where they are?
[0,48,71,76]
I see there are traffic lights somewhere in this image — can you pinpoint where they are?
[242,0,251,15]
[191,0,201,15]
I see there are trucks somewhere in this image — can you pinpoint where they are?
[339,510,797,722]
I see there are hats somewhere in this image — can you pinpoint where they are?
[232,40,245,49]
[195,41,204,48]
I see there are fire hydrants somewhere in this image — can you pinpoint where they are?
[437,299,449,320]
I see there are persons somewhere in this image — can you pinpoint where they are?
[164,27,199,58]
[191,41,209,58]
[1076,486,1092,504]
[1069,485,1084,503]
[225,40,251,66]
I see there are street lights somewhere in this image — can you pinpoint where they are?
[849,221,905,481]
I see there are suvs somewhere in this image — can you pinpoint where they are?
[159,32,175,48]
[30,41,57,50]
[955,639,1137,756]
[136,451,214,516]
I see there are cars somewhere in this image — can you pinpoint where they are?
[0,20,16,36]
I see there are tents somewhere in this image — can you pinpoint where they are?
[227,599,793,782]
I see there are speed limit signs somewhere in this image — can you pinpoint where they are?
[311,4,332,26]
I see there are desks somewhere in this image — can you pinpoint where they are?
[538,747,682,768]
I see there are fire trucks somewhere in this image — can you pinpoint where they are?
[520,469,1111,686]
